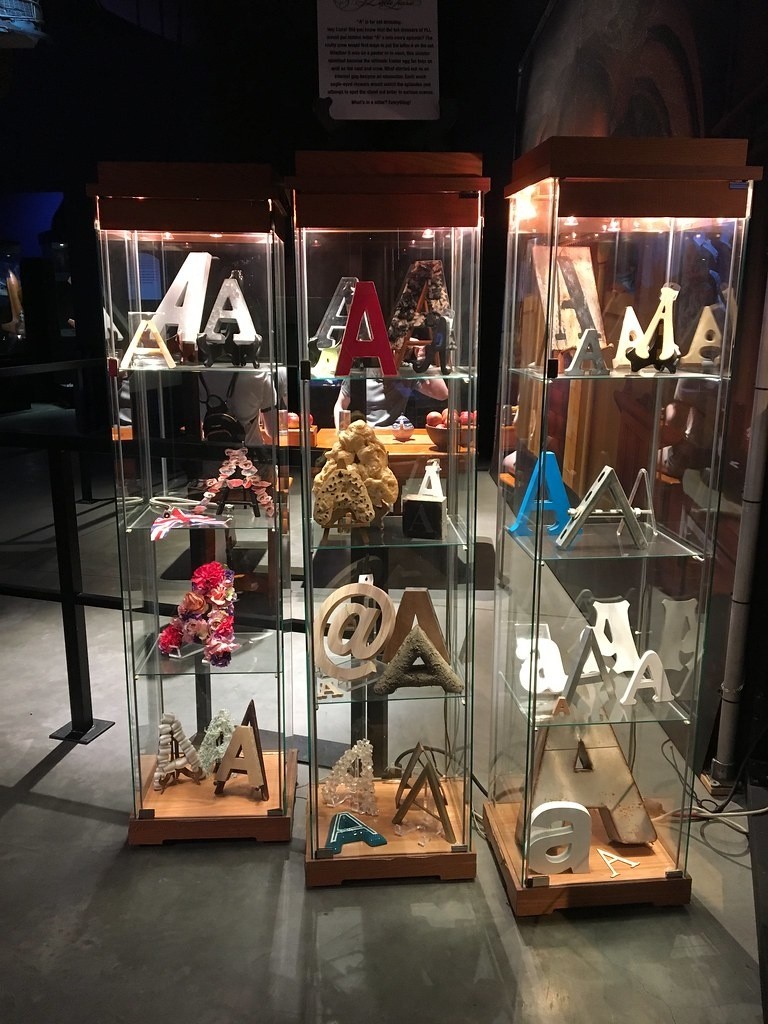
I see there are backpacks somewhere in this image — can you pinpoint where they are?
[196,373,255,443]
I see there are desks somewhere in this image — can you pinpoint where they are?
[112,426,476,516]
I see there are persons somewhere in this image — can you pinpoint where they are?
[199,366,278,486]
[333,335,449,430]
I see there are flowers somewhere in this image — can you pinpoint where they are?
[158,561,242,668]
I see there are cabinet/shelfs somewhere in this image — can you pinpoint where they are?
[292,148,490,890]
[599,391,749,777]
[88,159,299,845]
[481,136,763,924]
